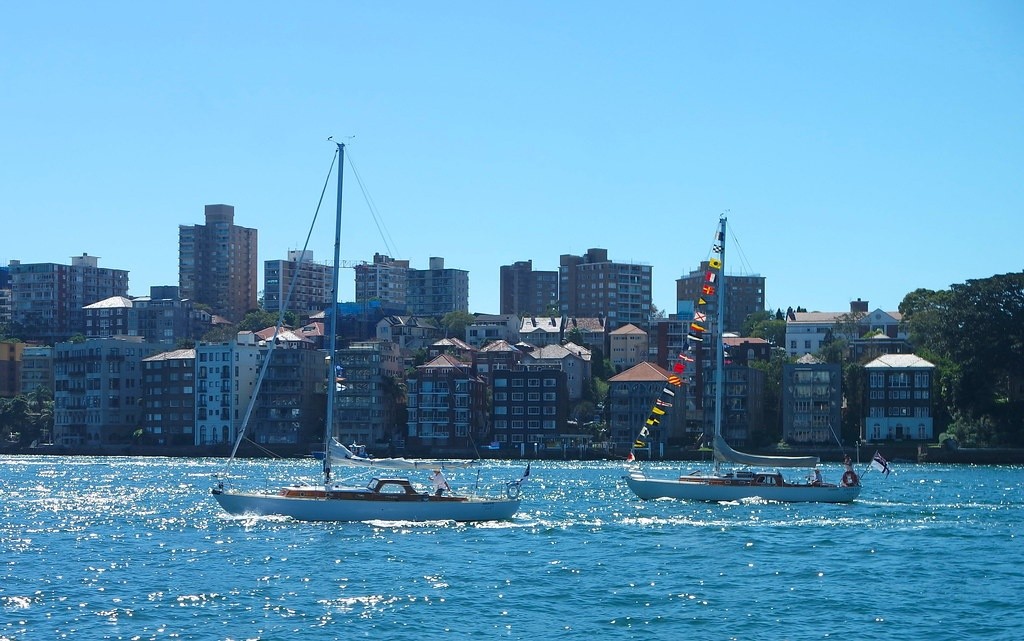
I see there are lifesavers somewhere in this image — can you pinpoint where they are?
[842,471,858,487]
[506,481,520,499]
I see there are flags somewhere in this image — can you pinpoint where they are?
[690,323,706,332]
[872,452,891,480]
[712,243,724,254]
[678,353,694,362]
[704,270,715,282]
[708,257,722,269]
[713,231,724,241]
[702,284,714,294]
[687,333,703,342]
[682,342,694,353]
[694,311,706,323]
[627,362,685,462]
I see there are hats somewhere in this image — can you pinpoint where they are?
[845,454,848,456]
[433,468,440,471]
[815,470,819,472]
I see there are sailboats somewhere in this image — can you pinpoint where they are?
[620,207,865,503]
[210,133,532,524]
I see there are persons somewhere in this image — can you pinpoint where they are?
[842,454,859,486]
[813,469,822,487]
[428,468,450,497]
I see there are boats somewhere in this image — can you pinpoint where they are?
[310,439,376,462]
